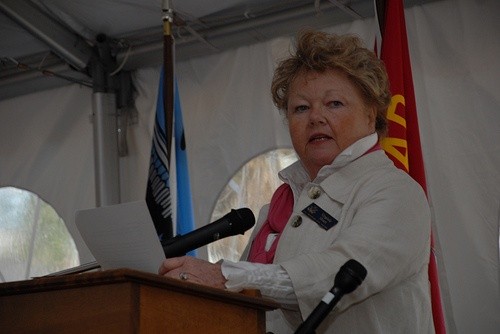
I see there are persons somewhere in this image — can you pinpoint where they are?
[159,31,436,334]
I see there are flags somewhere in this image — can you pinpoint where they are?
[144,34,197,258]
[375,0,447,334]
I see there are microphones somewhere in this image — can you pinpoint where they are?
[293,260,370,334]
[160,206,255,258]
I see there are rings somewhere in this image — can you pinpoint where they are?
[179,272,188,280]
